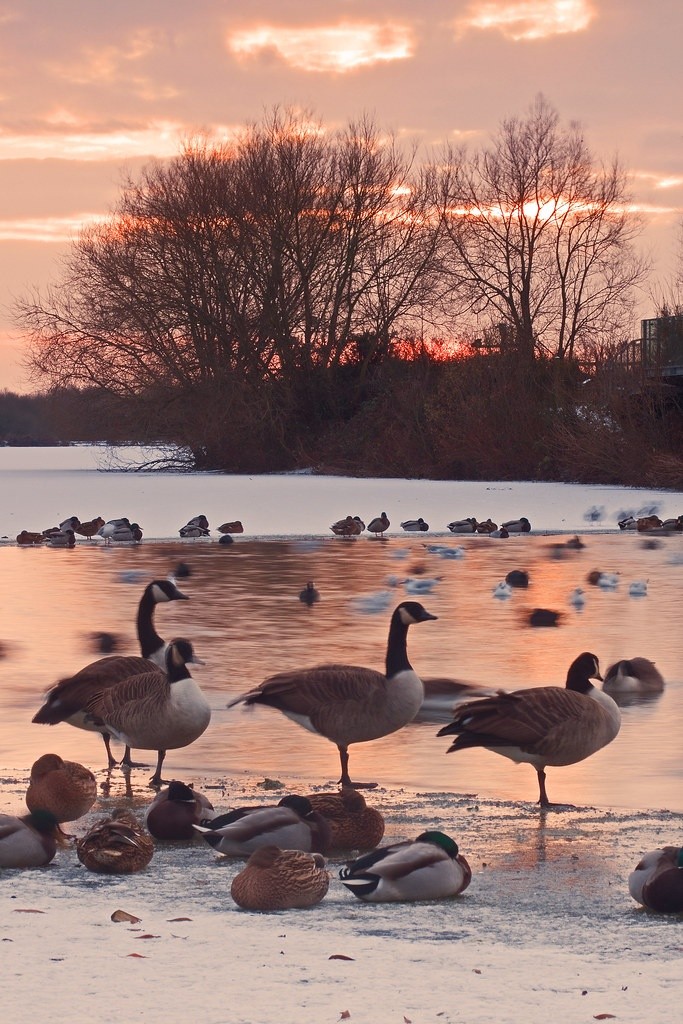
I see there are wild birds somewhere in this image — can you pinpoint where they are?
[29,559,195,768]
[367,511,390,538]
[76,808,154,874]
[305,788,385,857]
[335,831,471,904]
[546,534,683,604]
[230,845,329,911]
[25,754,97,842]
[144,781,218,842]
[16,516,146,546]
[628,846,683,914]
[617,515,683,532]
[329,515,366,539]
[357,542,467,613]
[602,657,666,695]
[84,637,212,786]
[399,517,430,532]
[434,652,622,809]
[493,568,565,626]
[224,601,508,789]
[299,581,320,605]
[447,517,531,539]
[191,794,330,858]
[178,514,211,538]
[215,520,244,543]
[0,809,76,868]
